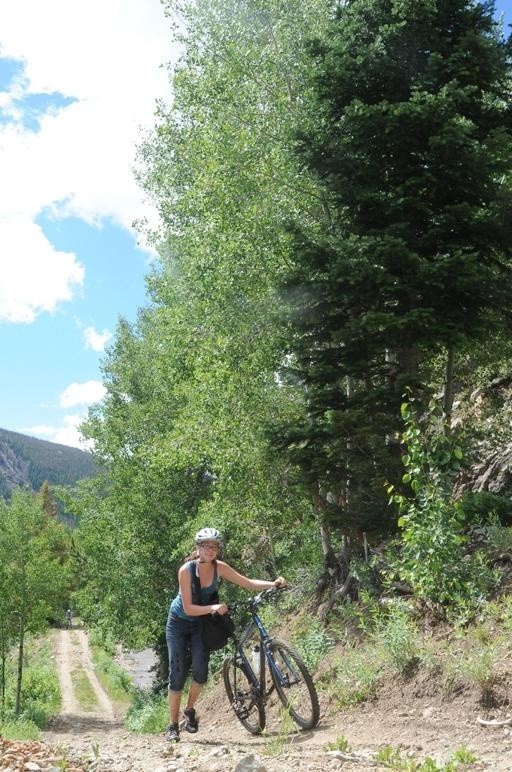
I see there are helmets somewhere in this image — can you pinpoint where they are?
[195,528,224,544]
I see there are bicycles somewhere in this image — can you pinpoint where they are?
[210,585,320,735]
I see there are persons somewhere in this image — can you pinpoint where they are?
[164,526,287,743]
[65,609,73,630]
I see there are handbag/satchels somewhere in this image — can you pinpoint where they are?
[201,611,235,650]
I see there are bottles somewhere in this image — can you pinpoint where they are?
[250,643,262,675]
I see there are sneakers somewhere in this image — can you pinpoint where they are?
[182,708,198,733]
[167,721,180,742]
